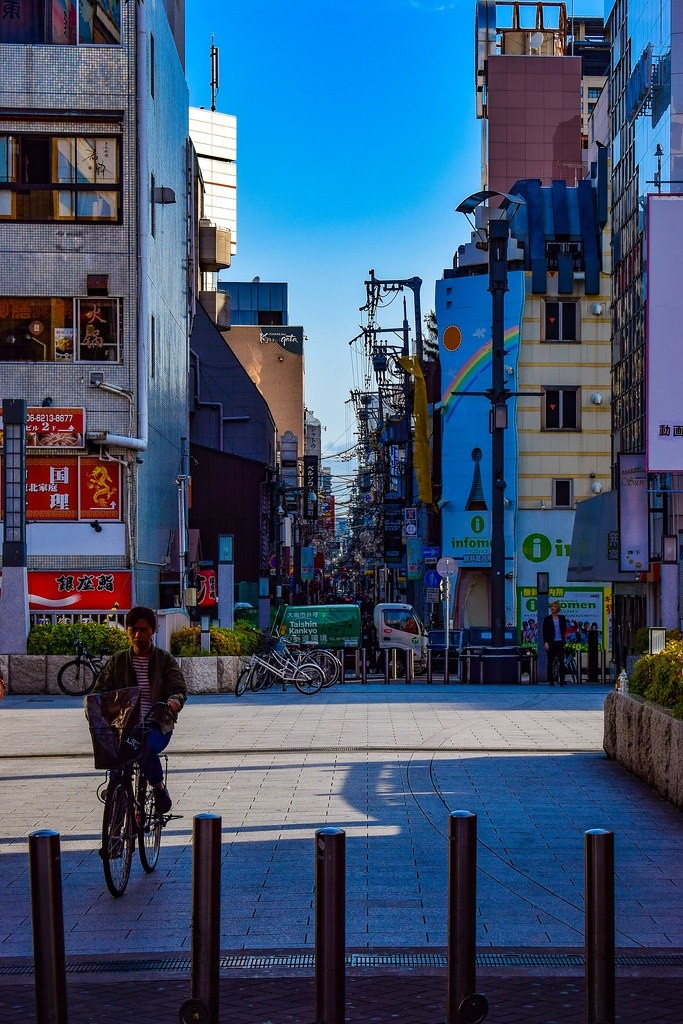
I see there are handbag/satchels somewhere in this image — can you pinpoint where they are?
[84,688,144,769]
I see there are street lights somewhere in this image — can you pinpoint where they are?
[450,190,545,686]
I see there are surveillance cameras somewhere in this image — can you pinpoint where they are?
[440,499,451,507]
[434,400,448,409]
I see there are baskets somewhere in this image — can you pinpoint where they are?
[276,640,286,655]
[90,726,146,770]
[256,636,275,655]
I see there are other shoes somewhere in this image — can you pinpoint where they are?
[549,682,554,686]
[560,681,567,686]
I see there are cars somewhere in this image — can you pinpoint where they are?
[428,628,463,674]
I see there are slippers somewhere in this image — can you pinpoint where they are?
[154,786,171,814]
[99,845,123,859]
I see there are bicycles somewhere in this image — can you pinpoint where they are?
[57,636,116,696]
[551,636,579,683]
[234,626,344,698]
[97,698,185,898]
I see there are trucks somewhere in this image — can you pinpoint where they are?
[270,602,429,679]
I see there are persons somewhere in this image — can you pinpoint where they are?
[542,601,567,687]
[318,593,375,617]
[521,617,604,644]
[85,606,188,859]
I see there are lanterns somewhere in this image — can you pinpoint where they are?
[195,569,216,607]
